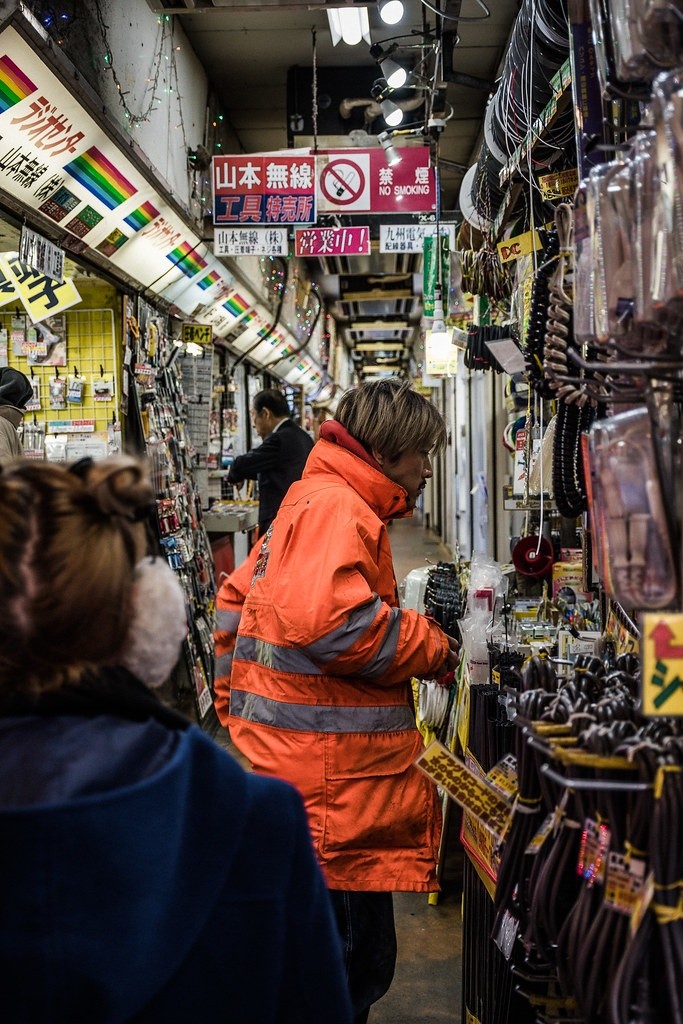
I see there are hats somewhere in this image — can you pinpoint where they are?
[0,367,33,409]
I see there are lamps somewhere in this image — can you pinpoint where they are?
[323,0,438,166]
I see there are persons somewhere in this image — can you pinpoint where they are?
[0,365,32,463]
[214,377,460,1023]
[222,391,315,541]
[1,459,354,1024]
